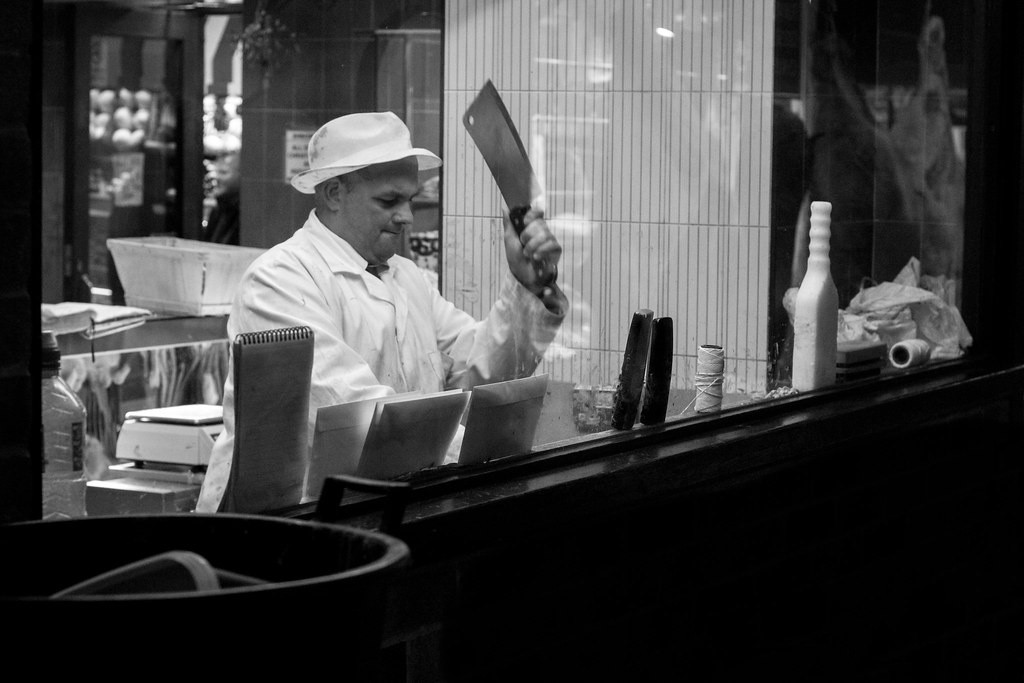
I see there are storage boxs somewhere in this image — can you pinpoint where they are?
[105,232,270,303]
[123,292,230,317]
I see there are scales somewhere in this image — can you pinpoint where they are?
[113,402,227,474]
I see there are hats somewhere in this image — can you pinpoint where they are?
[291,112,445,195]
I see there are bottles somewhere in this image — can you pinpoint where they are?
[40,330,88,520]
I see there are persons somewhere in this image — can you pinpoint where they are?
[189,110,567,527]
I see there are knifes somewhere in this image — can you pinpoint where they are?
[461,77,558,288]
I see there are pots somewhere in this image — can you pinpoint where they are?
[0,473,408,683]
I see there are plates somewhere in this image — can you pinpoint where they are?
[50,549,221,598]
